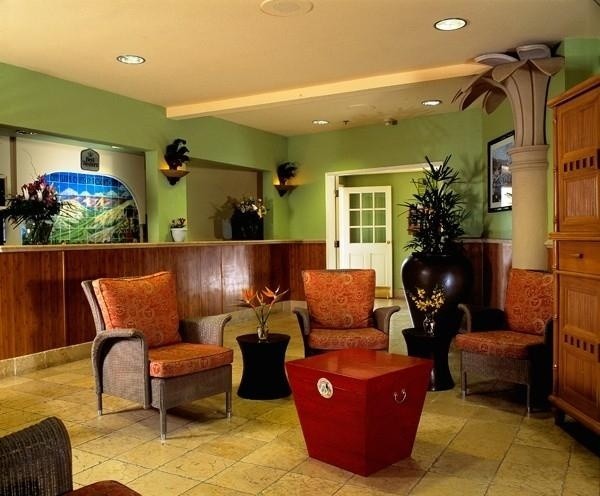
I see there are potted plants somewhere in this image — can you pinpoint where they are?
[170,217,188,242]
[395,153,473,337]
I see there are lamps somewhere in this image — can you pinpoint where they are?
[159,138,190,185]
[273,160,299,197]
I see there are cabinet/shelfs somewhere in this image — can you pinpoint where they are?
[546,74,600,436]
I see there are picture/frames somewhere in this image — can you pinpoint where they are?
[487,129,515,213]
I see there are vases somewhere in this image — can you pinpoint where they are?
[29,216,53,245]
[231,216,263,240]
[422,313,436,334]
[257,325,269,340]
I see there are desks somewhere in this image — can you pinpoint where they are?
[236,333,292,400]
[401,328,455,391]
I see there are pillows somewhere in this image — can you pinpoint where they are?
[301,269,376,330]
[504,268,553,336]
[92,271,183,349]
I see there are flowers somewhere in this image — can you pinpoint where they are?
[233,194,270,220]
[232,283,289,325]
[403,280,448,313]
[5,174,75,230]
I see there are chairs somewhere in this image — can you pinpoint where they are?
[80,271,234,443]
[0,416,142,496]
[454,268,553,413]
[292,269,400,358]
